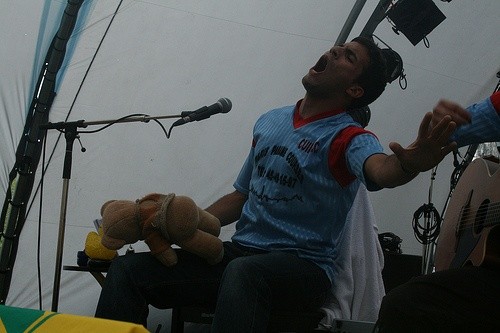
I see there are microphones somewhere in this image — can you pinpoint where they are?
[173,97,232,126]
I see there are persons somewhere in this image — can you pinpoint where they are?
[84,35,456,333]
[375,90,500,333]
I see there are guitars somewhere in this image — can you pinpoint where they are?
[432,157,499,278]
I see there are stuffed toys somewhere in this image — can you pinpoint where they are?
[99,191,225,267]
